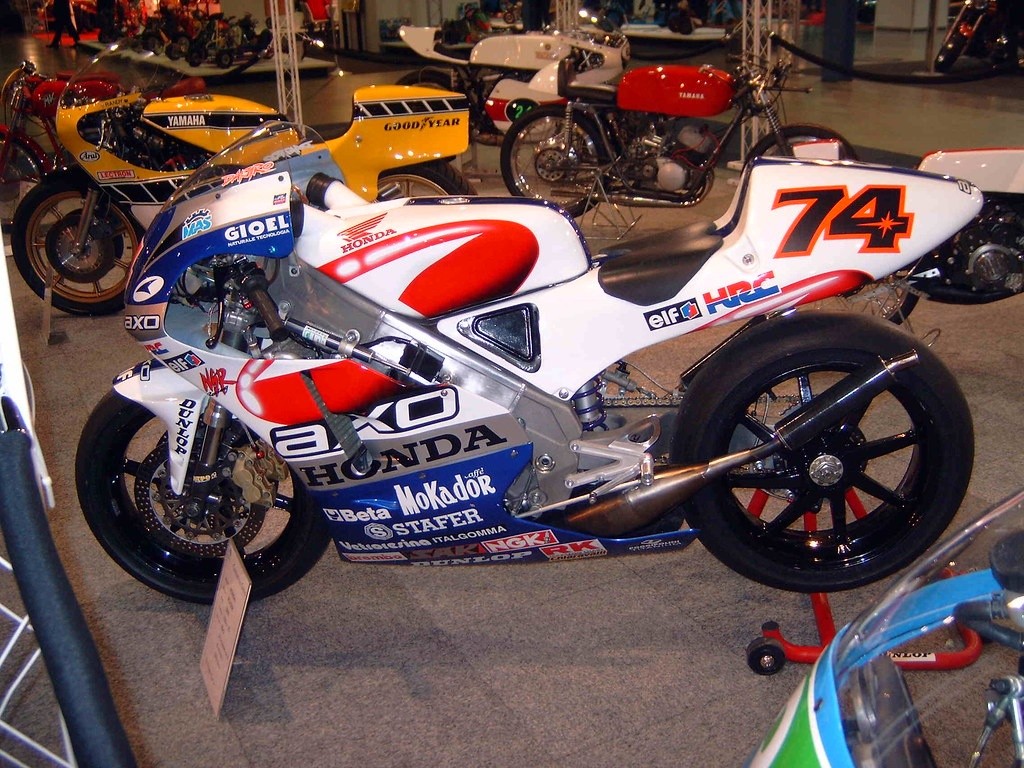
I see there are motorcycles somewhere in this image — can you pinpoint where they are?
[2,0,1024,768]
